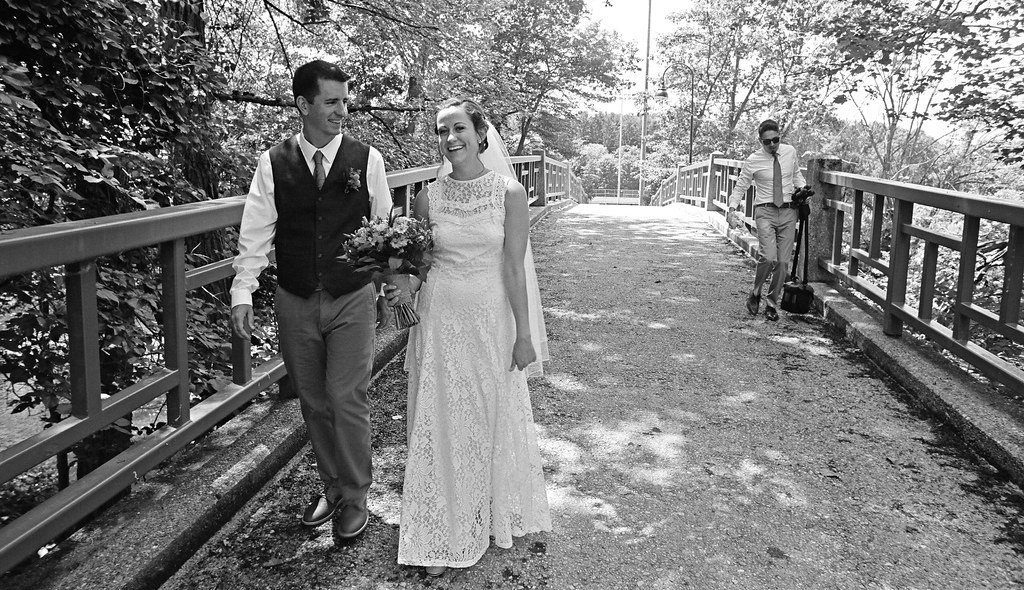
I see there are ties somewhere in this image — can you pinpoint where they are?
[312,151,326,193]
[772,152,783,208]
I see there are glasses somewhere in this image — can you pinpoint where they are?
[760,136,780,145]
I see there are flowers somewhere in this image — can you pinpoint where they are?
[344,167,361,193]
[728,211,746,230]
[336,207,439,331]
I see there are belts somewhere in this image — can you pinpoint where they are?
[756,203,795,209]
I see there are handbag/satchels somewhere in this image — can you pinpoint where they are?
[780,279,814,315]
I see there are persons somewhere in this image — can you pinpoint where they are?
[383,98,539,577]
[727,119,807,321]
[229,61,394,538]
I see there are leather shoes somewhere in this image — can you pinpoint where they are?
[302,488,344,526]
[337,493,369,539]
[746,289,761,316]
[764,306,779,321]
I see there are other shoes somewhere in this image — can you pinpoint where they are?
[426,566,447,576]
